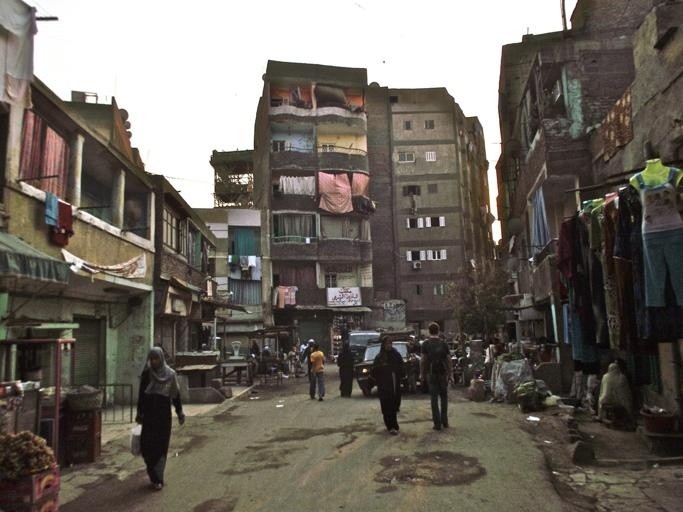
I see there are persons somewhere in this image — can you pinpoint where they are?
[133,346,185,490]
[249,336,317,378]
[594,360,634,427]
[305,344,326,401]
[484,341,497,377]
[370,334,404,435]
[416,321,454,430]
[336,340,355,397]
[558,158,683,348]
[405,333,420,356]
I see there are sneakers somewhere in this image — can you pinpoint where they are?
[388,427,400,436]
[149,478,164,490]
[431,423,450,431]
[310,393,323,401]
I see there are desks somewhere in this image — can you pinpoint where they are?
[178,364,216,387]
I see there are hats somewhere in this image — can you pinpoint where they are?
[308,339,315,342]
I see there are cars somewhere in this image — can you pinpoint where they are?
[348,330,424,396]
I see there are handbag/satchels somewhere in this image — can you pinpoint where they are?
[129,421,142,458]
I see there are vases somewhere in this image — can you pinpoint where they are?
[639,409,677,432]
